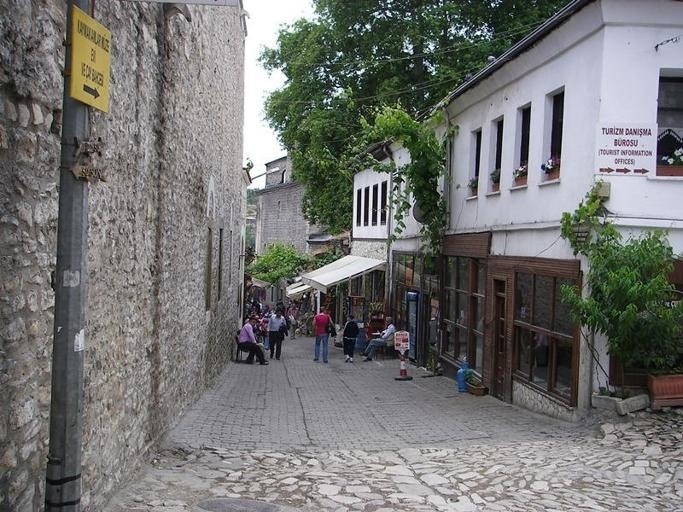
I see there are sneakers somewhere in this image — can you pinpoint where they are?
[247,353,280,365]
[314,352,371,363]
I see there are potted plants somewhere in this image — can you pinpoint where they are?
[466,376,486,395]
[636,302,683,409]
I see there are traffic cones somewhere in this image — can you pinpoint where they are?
[394,355,414,381]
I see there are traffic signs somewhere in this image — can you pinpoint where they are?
[70,3,111,113]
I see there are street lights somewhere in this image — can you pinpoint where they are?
[251,167,280,183]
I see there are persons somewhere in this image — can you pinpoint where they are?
[239,295,300,365]
[312,306,337,362]
[342,314,360,364]
[525,323,549,364]
[359,316,397,361]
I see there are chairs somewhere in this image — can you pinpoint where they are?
[375,327,400,359]
[235,335,258,362]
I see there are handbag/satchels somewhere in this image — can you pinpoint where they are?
[331,328,336,337]
[327,323,332,333]
